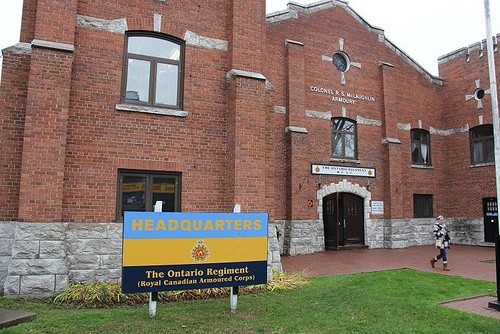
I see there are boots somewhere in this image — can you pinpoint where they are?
[443,261,450,271]
[431,257,437,267]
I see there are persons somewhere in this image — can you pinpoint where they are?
[430,215,451,271]
